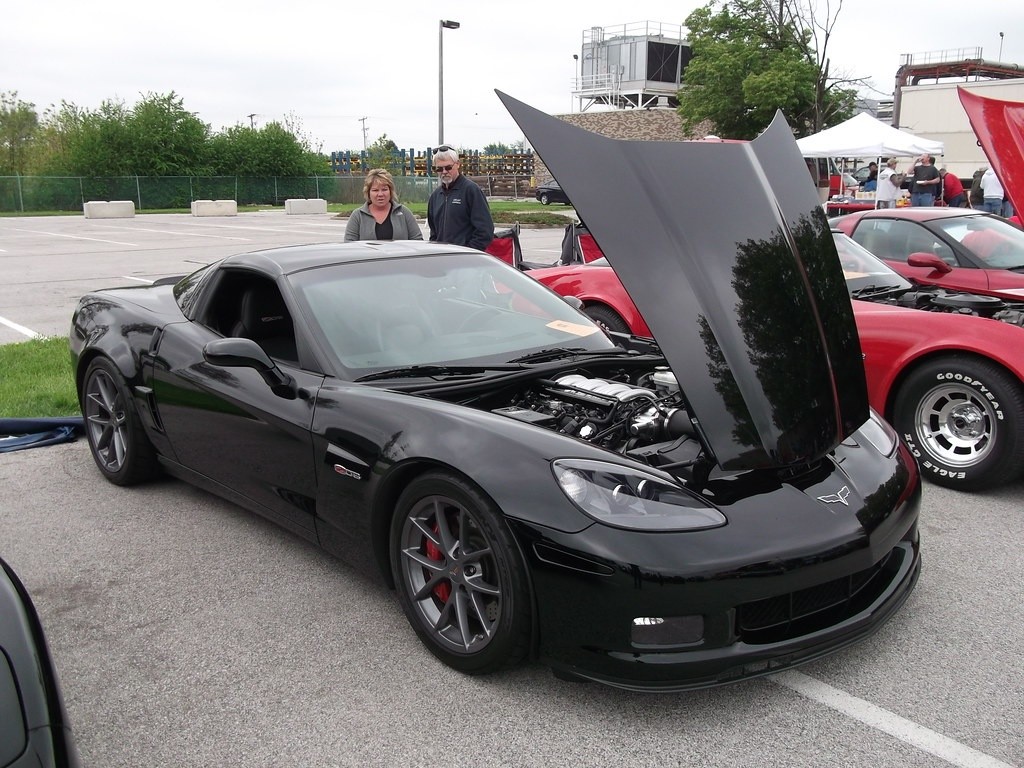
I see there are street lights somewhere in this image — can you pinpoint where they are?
[999,31,1005,64]
[247,115,256,132]
[574,54,579,90]
[438,18,462,143]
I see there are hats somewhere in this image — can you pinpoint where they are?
[887,158,898,167]
[866,162,876,168]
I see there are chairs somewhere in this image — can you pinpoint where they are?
[863,228,893,260]
[905,229,937,260]
[232,287,298,363]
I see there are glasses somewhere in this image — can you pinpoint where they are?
[432,146,455,156]
[435,161,455,172]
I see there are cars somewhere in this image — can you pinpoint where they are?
[852,163,888,186]
[536,180,571,206]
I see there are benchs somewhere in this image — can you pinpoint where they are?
[285,198,328,213]
[191,199,238,216]
[83,200,136,218]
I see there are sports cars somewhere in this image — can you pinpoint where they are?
[518,85,1024,493]
[69,85,924,696]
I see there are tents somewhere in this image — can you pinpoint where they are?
[795,112,944,217]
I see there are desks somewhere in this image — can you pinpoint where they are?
[827,200,948,217]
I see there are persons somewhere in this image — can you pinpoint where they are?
[343,169,424,243]
[427,144,494,252]
[906,153,942,207]
[876,157,907,209]
[969,166,1013,218]
[859,161,883,192]
[939,168,967,207]
